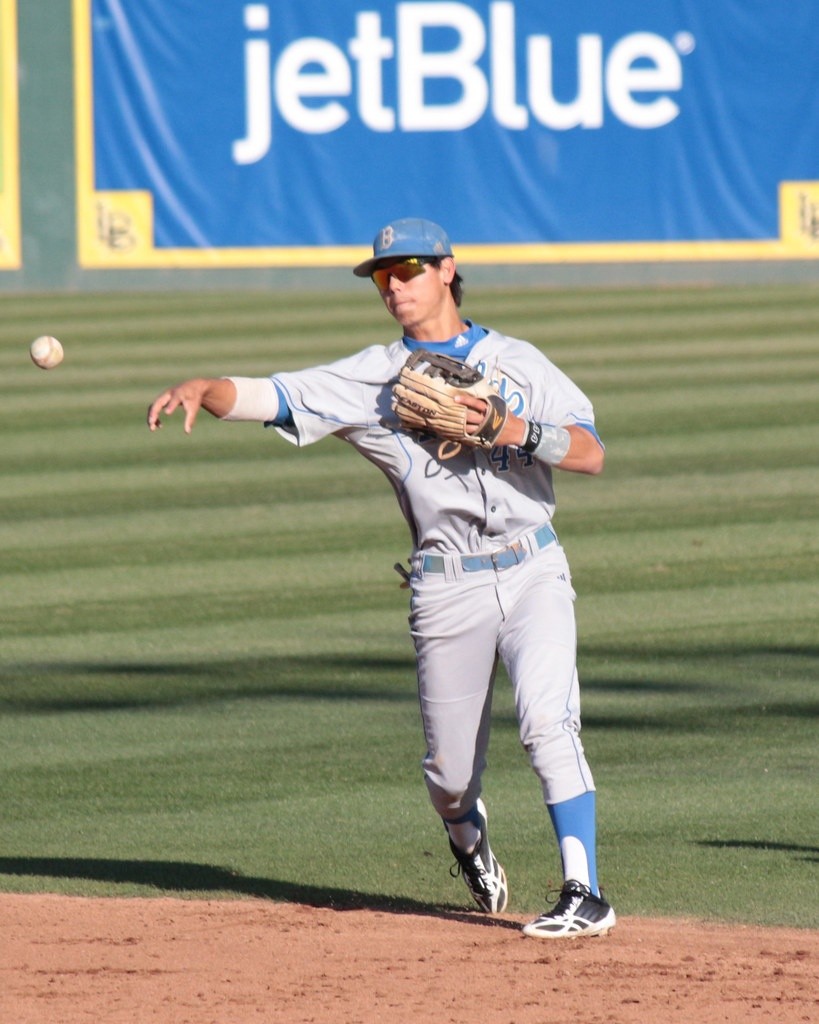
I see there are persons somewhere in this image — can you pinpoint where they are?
[148,215,616,938]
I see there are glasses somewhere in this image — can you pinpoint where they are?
[370,258,436,293]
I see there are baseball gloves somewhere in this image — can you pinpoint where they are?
[392,347,509,460]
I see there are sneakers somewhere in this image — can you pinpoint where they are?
[443,798,508,914]
[522,879,616,938]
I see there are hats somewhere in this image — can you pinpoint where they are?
[353,218,453,277]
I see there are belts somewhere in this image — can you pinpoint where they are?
[423,526,555,573]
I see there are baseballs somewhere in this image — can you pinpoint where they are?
[29,334,65,370]
